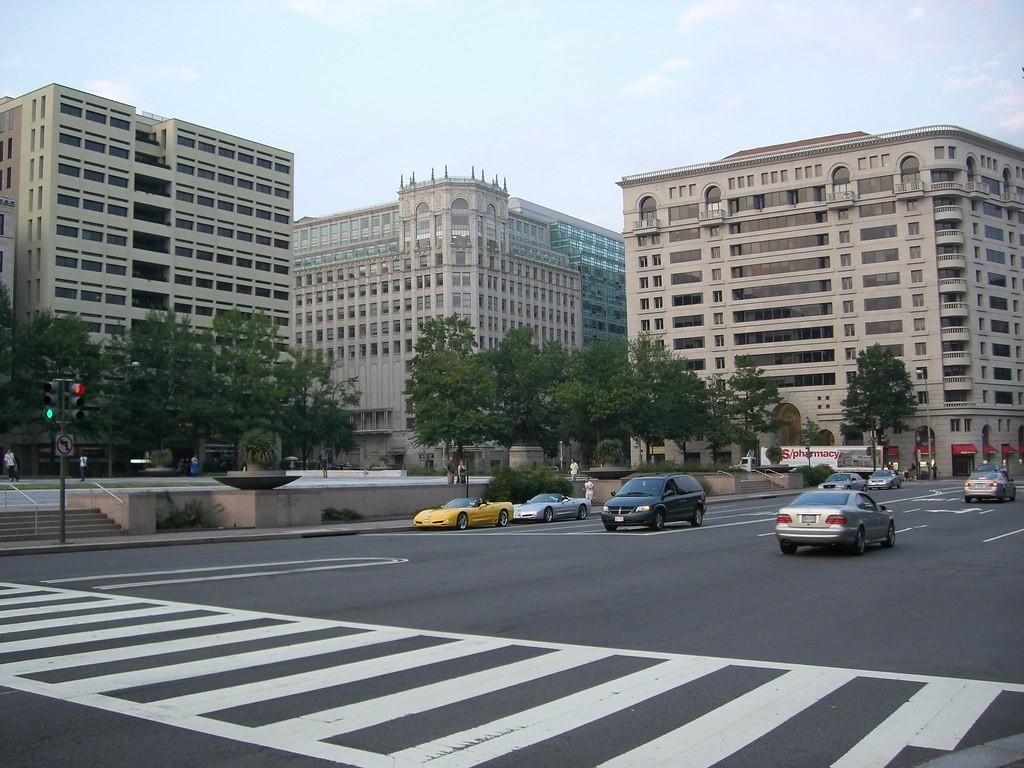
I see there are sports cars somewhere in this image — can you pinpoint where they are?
[509,493,592,524]
[413,498,515,531]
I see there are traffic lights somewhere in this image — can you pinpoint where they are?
[65,378,85,423]
[43,378,63,423]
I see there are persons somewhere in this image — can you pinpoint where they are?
[582,476,594,500]
[289,461,295,470]
[241,460,247,471]
[911,462,916,470]
[4,448,16,482]
[445,456,455,484]
[568,458,578,481]
[904,467,909,482]
[175,455,198,478]
[884,462,893,470]
[320,456,327,478]
[80,453,87,481]
[457,459,466,484]
[926,464,937,478]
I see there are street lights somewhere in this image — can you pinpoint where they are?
[559,440,563,468]
[109,362,142,476]
[916,369,933,481]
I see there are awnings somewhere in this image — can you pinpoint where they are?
[890,448,898,456]
[952,444,978,455]
[1002,446,1017,455]
[983,443,998,454]
[914,446,935,455]
[1019,445,1024,454]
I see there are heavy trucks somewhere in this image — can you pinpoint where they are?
[729,444,885,479]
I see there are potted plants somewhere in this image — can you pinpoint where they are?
[150,448,173,468]
[766,447,782,465]
[595,439,627,467]
[239,428,280,472]
[379,456,385,468]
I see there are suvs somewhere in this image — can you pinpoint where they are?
[600,475,707,533]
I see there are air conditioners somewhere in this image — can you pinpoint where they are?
[841,192,853,200]
[635,218,660,228]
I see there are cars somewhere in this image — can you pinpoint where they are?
[333,462,360,471]
[964,471,1016,503]
[970,461,1009,480]
[817,473,870,492]
[775,491,896,557]
[868,470,905,489]
